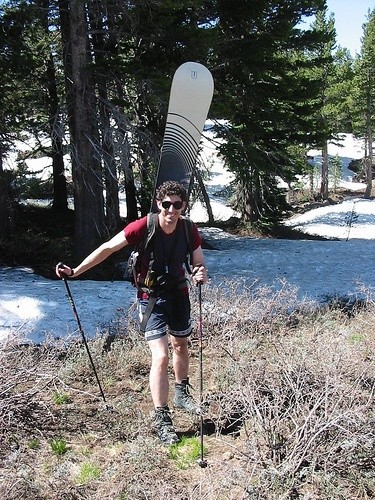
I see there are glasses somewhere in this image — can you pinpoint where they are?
[158,199,184,210]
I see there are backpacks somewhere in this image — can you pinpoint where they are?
[124,240,147,289]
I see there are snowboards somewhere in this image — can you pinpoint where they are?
[131,62,214,221]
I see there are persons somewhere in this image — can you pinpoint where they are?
[56,181,207,427]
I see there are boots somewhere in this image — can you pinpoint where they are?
[152,405,181,445]
[173,376,206,414]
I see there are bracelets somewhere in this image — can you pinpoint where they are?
[68,268,74,277]
